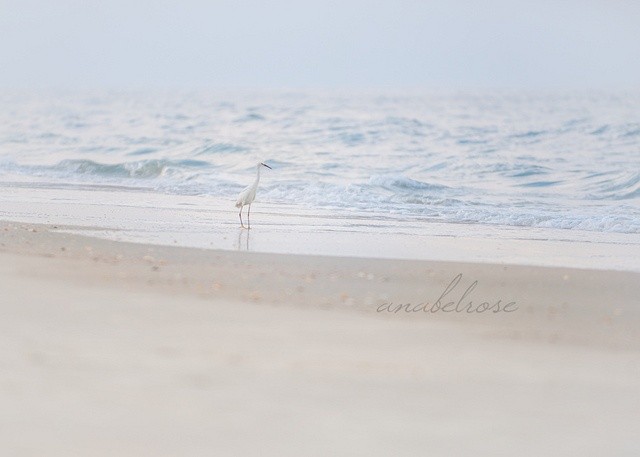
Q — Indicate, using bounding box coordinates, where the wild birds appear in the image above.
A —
[235,160,272,229]
